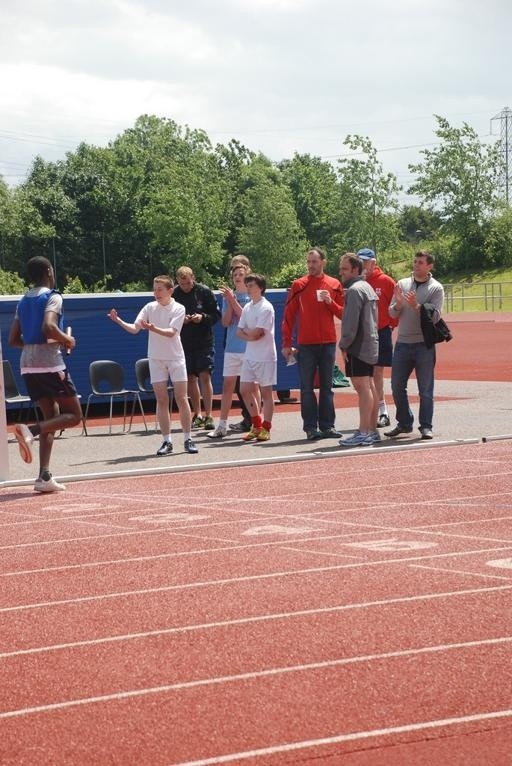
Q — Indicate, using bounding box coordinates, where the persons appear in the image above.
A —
[206,265,261,438]
[107,275,199,455]
[357,249,400,428]
[9,257,83,493]
[223,254,264,433]
[171,266,222,430]
[236,273,277,442]
[280,248,345,440]
[384,252,444,440]
[338,254,382,447]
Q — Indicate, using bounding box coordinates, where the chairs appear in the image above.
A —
[26,400,89,437]
[81,359,148,436]
[128,358,174,433]
[3,360,38,424]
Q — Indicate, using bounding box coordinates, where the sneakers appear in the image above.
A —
[422,428,433,439]
[157,441,173,455]
[244,424,263,441]
[205,415,215,430]
[14,424,34,463]
[185,440,198,453]
[230,421,249,430]
[308,429,324,440]
[384,424,412,436]
[258,428,271,440]
[192,416,204,429]
[323,426,342,437]
[206,426,227,438]
[340,432,374,446]
[377,414,391,427]
[34,475,66,493]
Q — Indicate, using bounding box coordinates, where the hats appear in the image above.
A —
[357,248,374,260]
